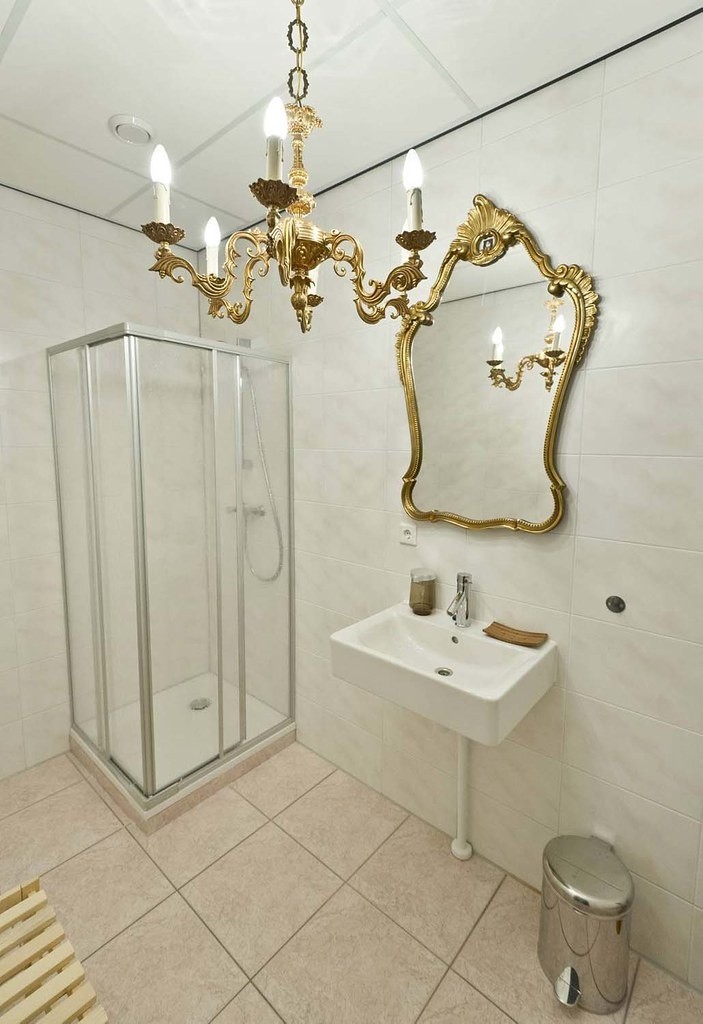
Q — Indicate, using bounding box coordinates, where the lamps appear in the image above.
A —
[142,1,434,334]
[486,294,569,392]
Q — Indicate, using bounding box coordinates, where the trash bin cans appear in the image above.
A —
[537,834,636,1016]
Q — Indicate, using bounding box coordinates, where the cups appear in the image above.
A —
[408,571,437,616]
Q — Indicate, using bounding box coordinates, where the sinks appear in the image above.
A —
[328,602,560,747]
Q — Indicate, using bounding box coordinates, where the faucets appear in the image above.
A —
[446,572,473,628]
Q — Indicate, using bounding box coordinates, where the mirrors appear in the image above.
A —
[397,193,601,534]
